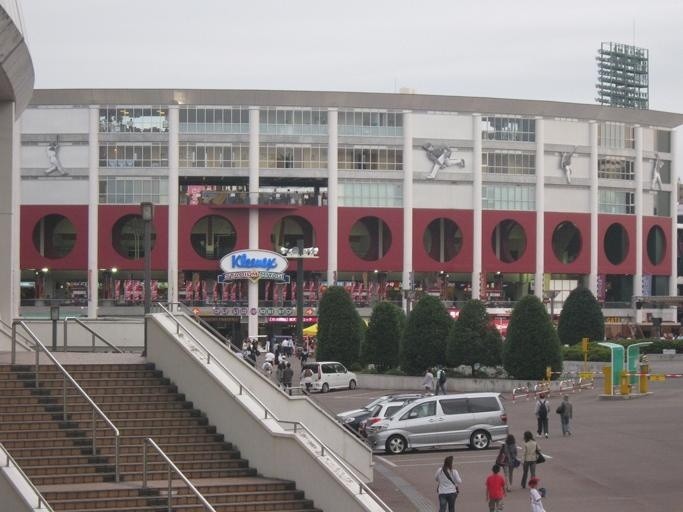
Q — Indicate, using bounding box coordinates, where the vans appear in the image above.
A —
[365,392,509,454]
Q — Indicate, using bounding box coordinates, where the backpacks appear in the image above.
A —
[440,370,446,384]
[539,400,547,418]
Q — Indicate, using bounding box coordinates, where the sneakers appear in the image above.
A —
[536,432,548,440]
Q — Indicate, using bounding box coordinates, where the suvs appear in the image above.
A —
[300,361,357,393]
[336,393,434,437]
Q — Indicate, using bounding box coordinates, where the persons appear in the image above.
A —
[435,365,448,394]
[534,392,550,439]
[527,480,545,512]
[501,433,518,491]
[434,455,463,511]
[235,337,313,395]
[561,394,573,436]
[420,368,433,392]
[520,429,540,488]
[484,464,508,511]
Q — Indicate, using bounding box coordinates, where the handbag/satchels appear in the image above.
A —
[495,444,508,466]
[536,450,546,464]
[556,406,565,414]
